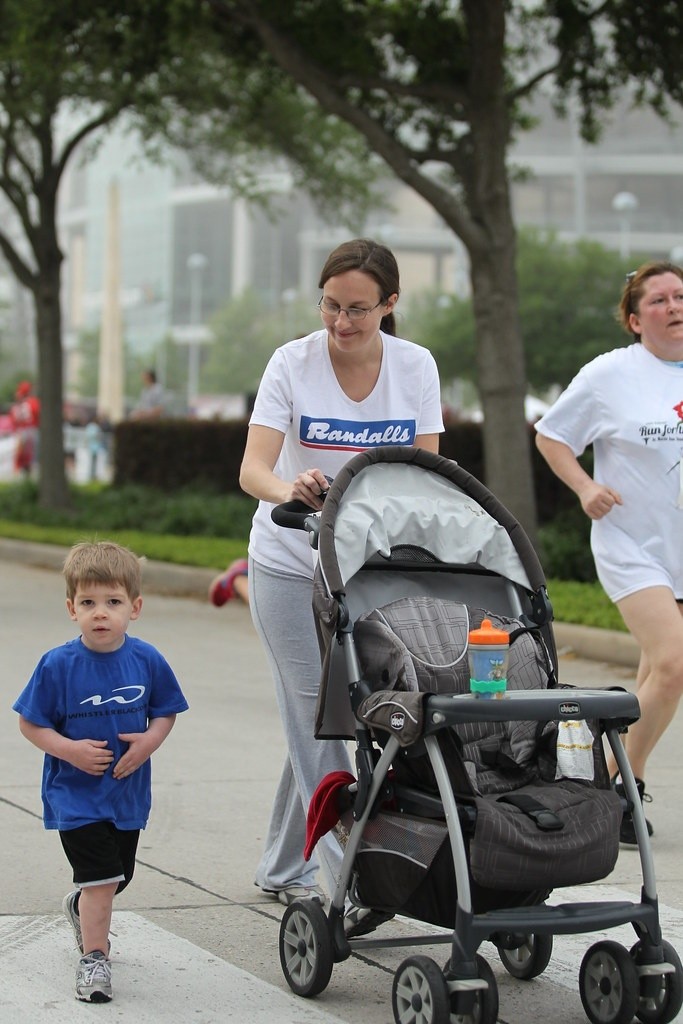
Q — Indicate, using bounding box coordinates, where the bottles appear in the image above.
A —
[467,619,509,701]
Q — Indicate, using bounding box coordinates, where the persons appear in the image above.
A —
[206,239,458,940]
[62,409,115,484]
[12,541,190,1003]
[533,264,683,849]
[10,379,39,481]
[129,366,169,418]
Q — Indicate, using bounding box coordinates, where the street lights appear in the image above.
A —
[189,251,205,416]
[613,188,638,258]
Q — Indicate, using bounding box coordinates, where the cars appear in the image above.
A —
[62,393,106,457]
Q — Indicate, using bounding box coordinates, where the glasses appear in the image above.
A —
[318,296,383,321]
[625,271,638,314]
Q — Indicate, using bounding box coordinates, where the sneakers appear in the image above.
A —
[610,770,653,849]
[342,903,397,939]
[75,949,113,1003]
[254,879,326,907]
[211,559,249,607]
[63,891,118,956]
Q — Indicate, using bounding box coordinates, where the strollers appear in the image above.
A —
[271,442,683,1024]
